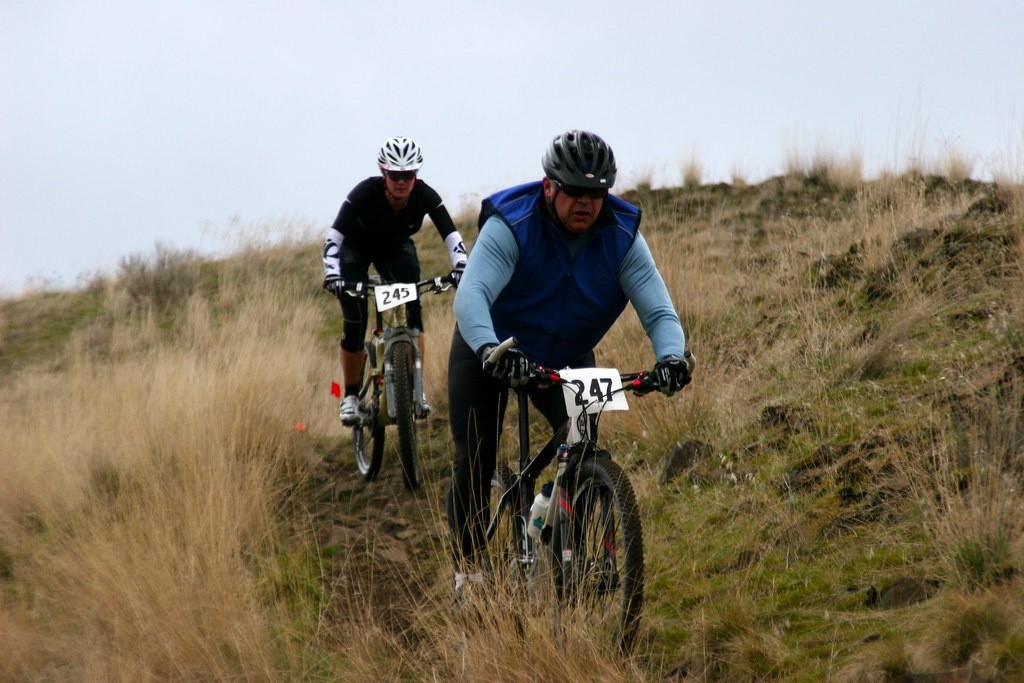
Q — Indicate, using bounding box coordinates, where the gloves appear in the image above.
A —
[449,266,465,284]
[323,275,345,297]
[633,357,689,399]
[480,346,530,389]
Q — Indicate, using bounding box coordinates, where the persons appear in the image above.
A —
[324,136,468,423]
[449,131,692,616]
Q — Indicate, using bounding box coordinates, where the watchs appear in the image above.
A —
[456,261,467,267]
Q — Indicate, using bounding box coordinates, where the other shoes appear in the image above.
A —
[565,561,619,590]
[451,577,488,624]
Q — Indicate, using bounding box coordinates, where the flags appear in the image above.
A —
[331,382,340,397]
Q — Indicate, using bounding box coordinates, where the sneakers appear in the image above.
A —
[339,394,359,421]
[419,392,431,417]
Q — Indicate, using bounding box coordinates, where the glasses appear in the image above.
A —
[551,177,608,199]
[385,171,417,182]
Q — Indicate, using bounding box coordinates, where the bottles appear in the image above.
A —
[528,481,554,539]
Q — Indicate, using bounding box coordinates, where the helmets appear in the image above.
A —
[377,136,422,173]
[541,128,616,189]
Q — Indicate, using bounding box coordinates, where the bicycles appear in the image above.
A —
[342,269,462,494]
[483,335,696,658]
